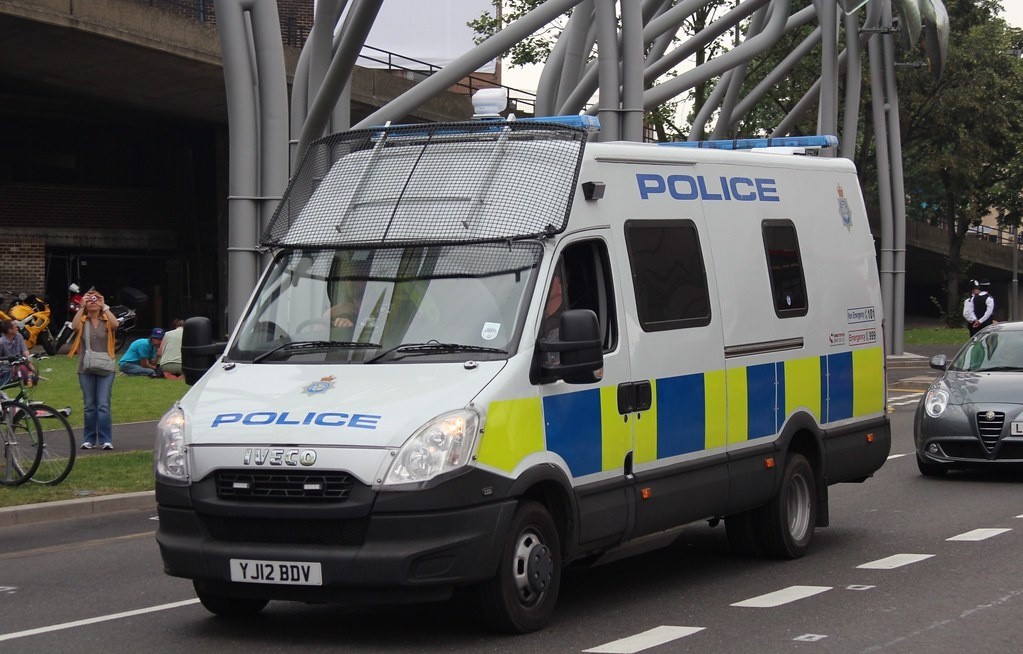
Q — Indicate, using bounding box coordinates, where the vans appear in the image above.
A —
[153,88,893,641]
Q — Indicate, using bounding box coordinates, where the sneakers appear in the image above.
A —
[80,442,96,449]
[102,442,114,449]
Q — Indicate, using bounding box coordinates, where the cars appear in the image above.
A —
[913,322,1022,478]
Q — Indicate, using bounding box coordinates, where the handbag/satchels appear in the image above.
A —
[82,350,114,376]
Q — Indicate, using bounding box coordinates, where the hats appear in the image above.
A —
[151,328,165,339]
[978,279,991,286]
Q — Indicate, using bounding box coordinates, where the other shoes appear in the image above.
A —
[178,376,184,380]
[164,372,177,380]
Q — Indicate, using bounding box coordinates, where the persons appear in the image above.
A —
[117,318,186,381]
[72,289,119,451]
[323,260,422,337]
[962,278,994,339]
[0,319,35,376]
[540,264,575,366]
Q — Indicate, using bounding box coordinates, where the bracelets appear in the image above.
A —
[80,305,85,308]
[102,305,110,312]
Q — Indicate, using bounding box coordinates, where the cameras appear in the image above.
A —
[89,296,97,302]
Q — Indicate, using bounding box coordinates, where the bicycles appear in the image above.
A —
[0,351,77,488]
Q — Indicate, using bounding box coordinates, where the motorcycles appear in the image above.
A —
[55,282,137,354]
[0,290,57,357]
